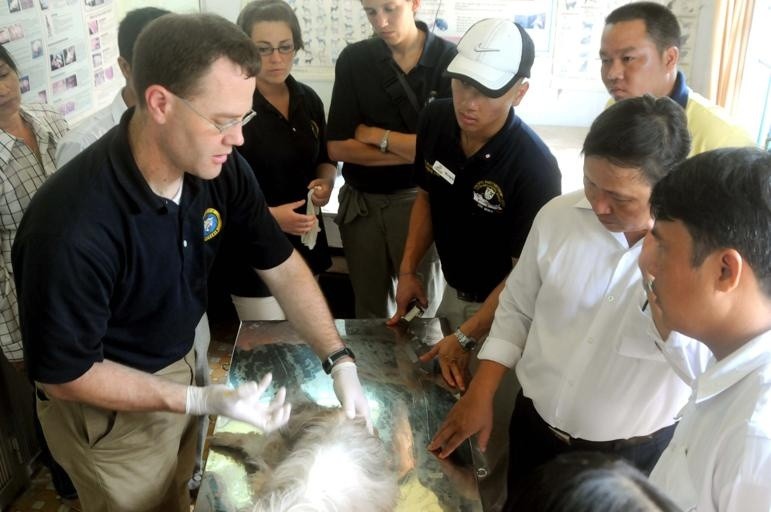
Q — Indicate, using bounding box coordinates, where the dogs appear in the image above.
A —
[206,402,401,512]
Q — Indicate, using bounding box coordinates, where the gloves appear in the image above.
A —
[330,362,373,434]
[185,372,291,433]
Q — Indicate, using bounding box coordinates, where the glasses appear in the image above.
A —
[180,98,257,133]
[256,44,294,56]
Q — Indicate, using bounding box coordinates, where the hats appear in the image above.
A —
[441,17,535,98]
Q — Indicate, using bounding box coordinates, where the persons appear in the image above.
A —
[1,0,771,512]
